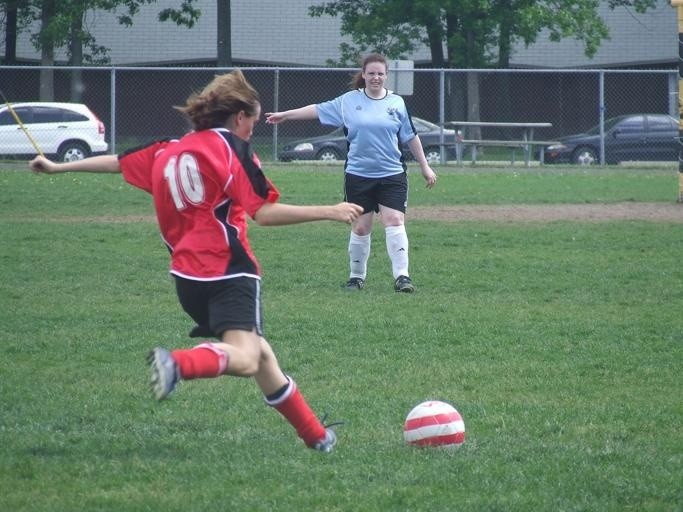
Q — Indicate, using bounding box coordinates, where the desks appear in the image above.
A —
[438,120,555,165]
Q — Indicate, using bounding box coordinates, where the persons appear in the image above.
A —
[28,66,364,456]
[264,54,437,293]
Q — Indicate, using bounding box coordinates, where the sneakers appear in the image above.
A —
[392,274,415,294]
[346,275,365,292]
[308,413,344,455]
[144,346,183,403]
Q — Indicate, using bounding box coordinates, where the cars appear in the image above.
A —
[0,102,108,163]
[535,113,680,166]
[279,113,463,162]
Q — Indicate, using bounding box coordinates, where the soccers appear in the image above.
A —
[403,401,466,450]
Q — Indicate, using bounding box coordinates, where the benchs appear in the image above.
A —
[428,137,559,167]
